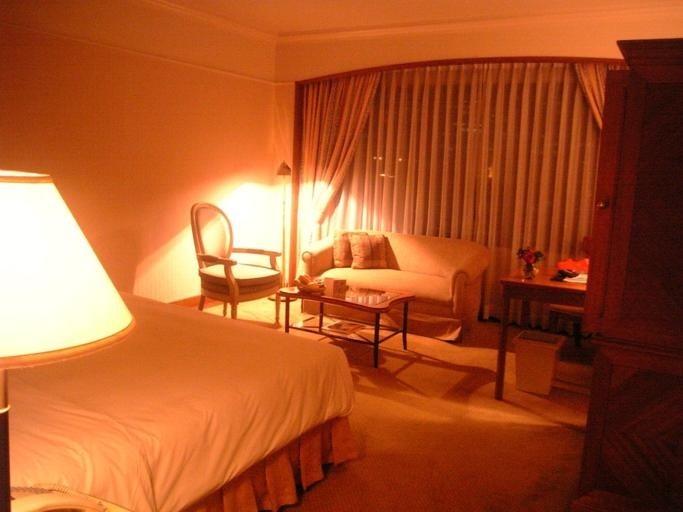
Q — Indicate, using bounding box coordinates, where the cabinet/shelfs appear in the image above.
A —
[577,34,682,505]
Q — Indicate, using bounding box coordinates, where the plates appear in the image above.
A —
[298,287,325,293]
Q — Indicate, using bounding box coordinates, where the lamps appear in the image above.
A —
[0,169,136,511]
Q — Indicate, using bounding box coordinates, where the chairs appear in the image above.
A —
[189,202,283,330]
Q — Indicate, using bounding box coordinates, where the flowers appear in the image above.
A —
[516,246,543,271]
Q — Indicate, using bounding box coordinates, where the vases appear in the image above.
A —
[520,264,539,280]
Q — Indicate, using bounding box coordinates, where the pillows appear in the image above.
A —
[330,230,387,269]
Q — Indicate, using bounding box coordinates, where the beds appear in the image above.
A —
[4,292,356,512]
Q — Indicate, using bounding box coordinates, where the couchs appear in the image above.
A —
[299,228,491,345]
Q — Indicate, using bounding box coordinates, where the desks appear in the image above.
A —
[494,267,586,401]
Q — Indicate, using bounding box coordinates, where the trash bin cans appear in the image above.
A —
[513,330,567,396]
